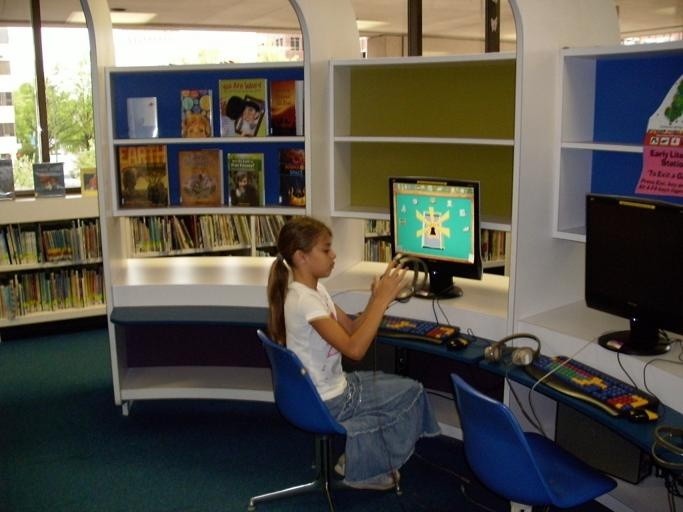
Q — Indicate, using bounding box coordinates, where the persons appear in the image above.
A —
[266,216,446,490]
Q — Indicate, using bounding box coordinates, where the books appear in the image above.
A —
[364,217,394,264]
[0,219,107,319]
[0,153,15,201]
[226,152,265,206]
[181,89,212,139]
[119,145,171,209]
[32,162,66,197]
[268,78,303,134]
[129,215,291,257]
[176,148,224,209]
[218,77,268,137]
[278,150,306,207]
[126,96,157,139]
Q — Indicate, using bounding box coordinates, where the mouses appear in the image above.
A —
[629,409,659,422]
[449,336,470,351]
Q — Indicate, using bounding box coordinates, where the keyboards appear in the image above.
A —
[350,311,460,346]
[533,356,659,417]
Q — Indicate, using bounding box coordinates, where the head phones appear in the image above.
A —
[379,253,427,303]
[484,333,541,366]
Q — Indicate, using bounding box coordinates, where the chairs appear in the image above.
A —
[246,329,404,512]
[449,371,618,512]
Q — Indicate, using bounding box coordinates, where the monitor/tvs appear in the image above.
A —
[585,193,683,356]
[389,176,482,299]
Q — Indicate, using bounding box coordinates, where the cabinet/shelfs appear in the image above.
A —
[104,61,306,217]
[328,51,523,234]
[0,196,510,344]
[549,41,683,244]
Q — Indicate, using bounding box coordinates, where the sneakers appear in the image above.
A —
[334,453,345,476]
[342,469,400,490]
